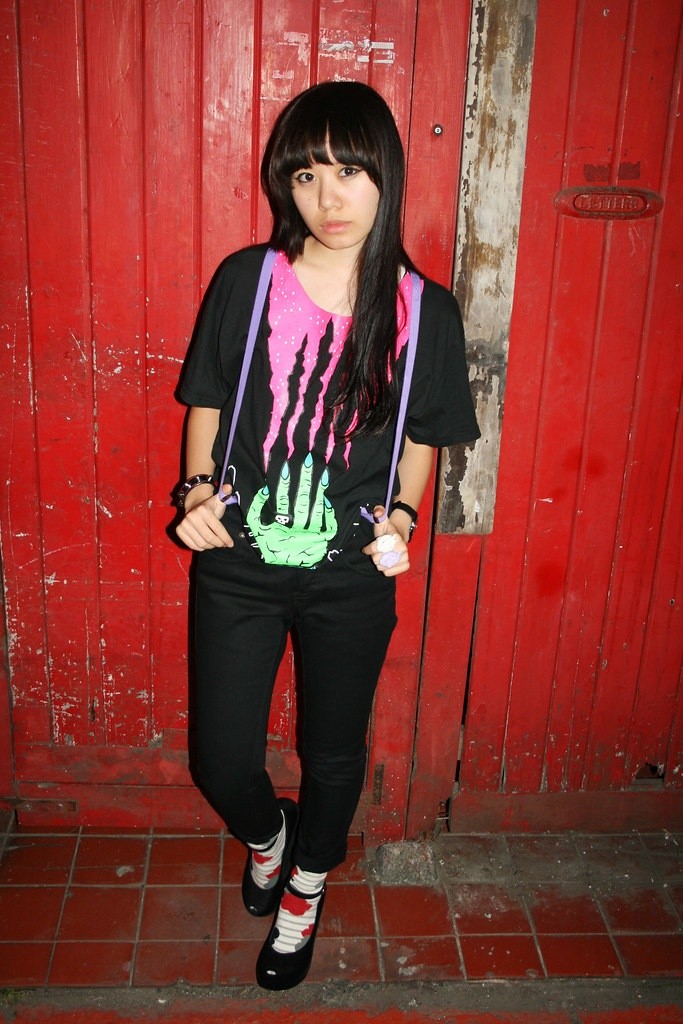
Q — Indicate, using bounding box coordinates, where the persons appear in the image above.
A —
[168,79,482,988]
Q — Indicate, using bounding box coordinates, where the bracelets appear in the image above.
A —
[174,473,219,509]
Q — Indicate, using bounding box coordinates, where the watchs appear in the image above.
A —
[383,499,420,541]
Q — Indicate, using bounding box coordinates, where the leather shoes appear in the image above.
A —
[241,797,326,990]
